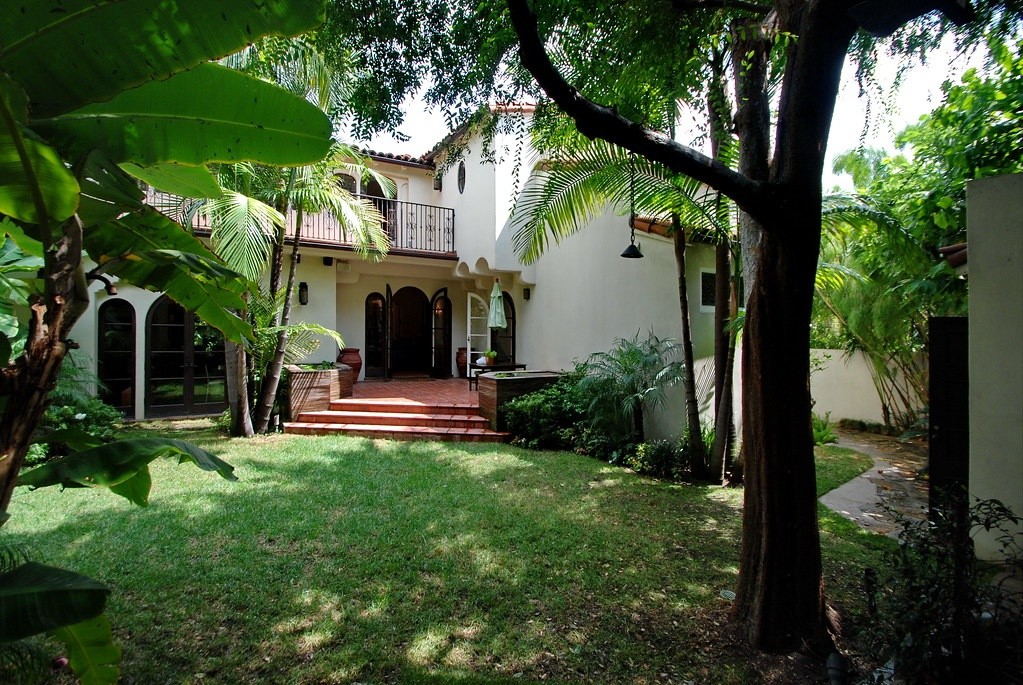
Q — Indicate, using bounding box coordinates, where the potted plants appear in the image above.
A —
[486,351,497,365]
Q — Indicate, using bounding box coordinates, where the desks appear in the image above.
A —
[469,361,526,391]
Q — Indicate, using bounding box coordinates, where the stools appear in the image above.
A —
[474,371,489,390]
